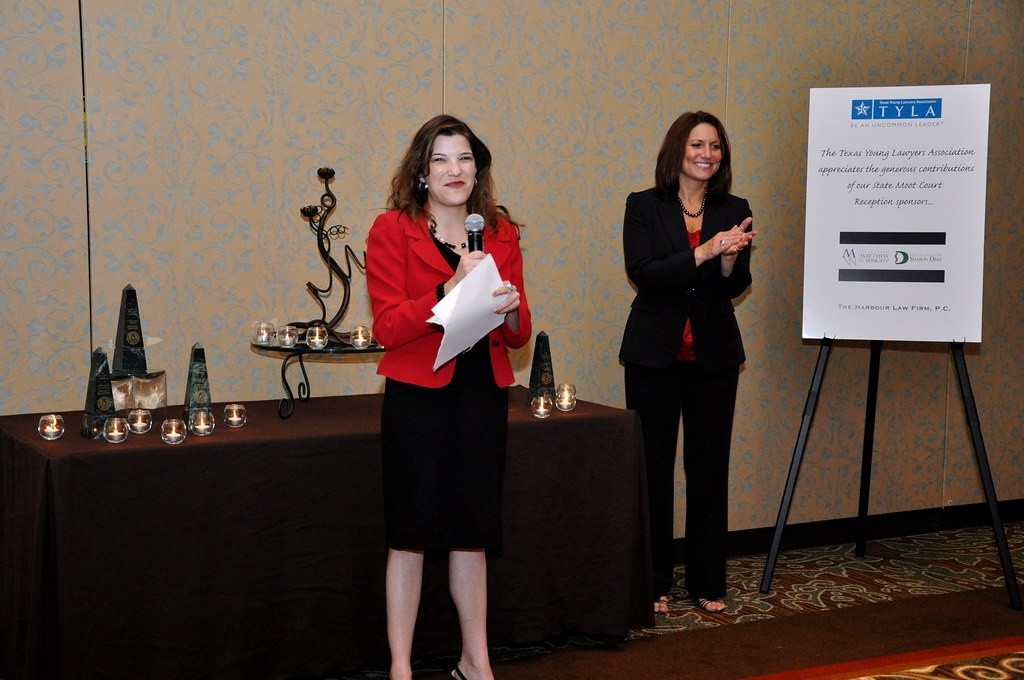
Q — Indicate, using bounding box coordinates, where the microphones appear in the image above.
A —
[464,214,485,253]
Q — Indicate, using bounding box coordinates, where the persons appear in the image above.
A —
[619,111,757,616]
[366,115,532,680]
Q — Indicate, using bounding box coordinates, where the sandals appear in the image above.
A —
[699,598,726,613]
[655,595,669,613]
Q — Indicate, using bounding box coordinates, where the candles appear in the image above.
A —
[127,410,152,433]
[103,418,128,443]
[277,326,298,347]
[161,419,188,445]
[189,412,215,436]
[253,322,275,344]
[350,326,371,349]
[532,396,552,418]
[556,391,577,412]
[223,404,246,427]
[37,414,65,439]
[556,384,576,399]
[307,326,329,349]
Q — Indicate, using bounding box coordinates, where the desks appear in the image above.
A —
[0,386,655,680]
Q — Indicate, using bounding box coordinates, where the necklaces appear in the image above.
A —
[678,192,707,217]
[427,222,469,248]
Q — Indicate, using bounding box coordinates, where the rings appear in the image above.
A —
[720,240,724,246]
[736,245,743,251]
[740,225,745,230]
[506,283,513,293]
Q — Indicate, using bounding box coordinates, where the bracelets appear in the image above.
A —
[437,282,445,302]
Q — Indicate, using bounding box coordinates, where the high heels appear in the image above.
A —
[450,660,467,680]
